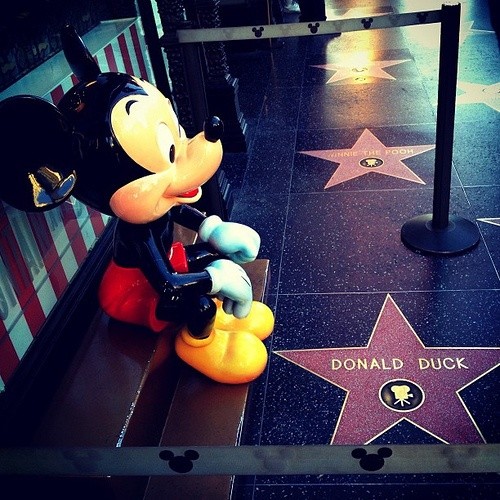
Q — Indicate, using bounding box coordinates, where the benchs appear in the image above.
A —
[43,257,272,500]
